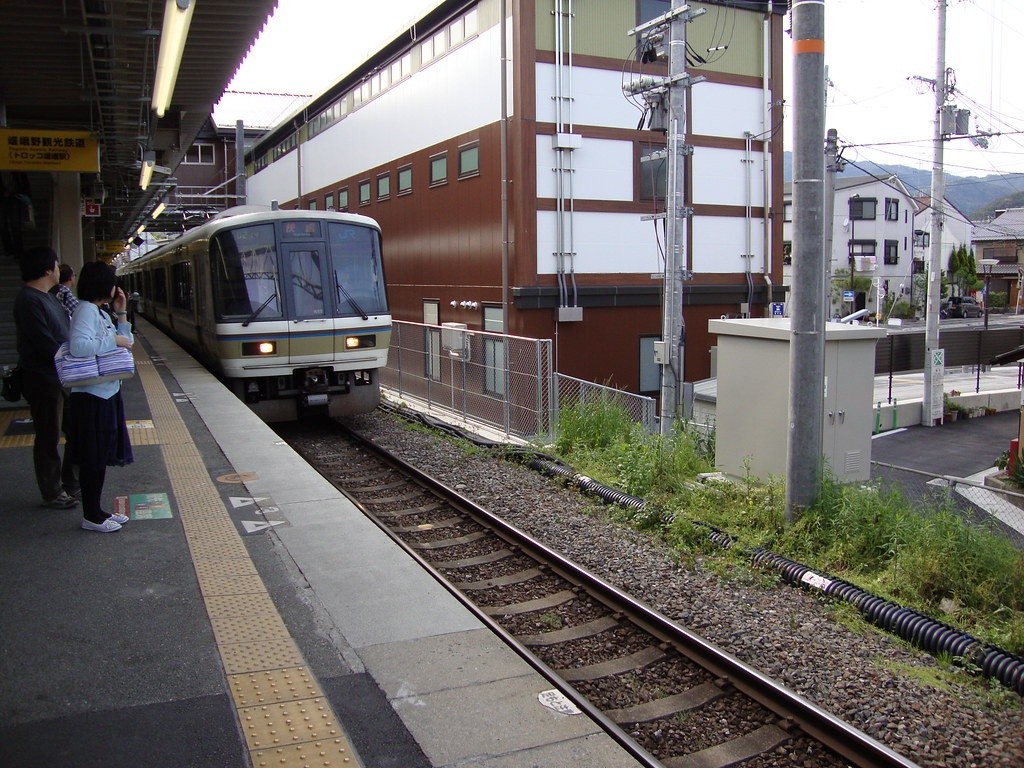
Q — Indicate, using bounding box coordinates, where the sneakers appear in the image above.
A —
[43,491,80,509]
[72,489,82,500]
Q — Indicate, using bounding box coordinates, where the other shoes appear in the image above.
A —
[82,518,122,532]
[109,513,129,523]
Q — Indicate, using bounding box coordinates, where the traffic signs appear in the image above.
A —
[843,291,854,301]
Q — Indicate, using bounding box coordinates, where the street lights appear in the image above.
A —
[978,259,1001,331]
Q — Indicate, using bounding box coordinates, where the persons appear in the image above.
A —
[14,247,140,531]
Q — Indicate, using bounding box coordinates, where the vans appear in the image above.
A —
[948,297,983,318]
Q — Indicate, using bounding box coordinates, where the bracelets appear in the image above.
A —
[116,311,127,315]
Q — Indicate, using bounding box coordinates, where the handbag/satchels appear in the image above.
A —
[1,368,19,402]
[53,305,135,387]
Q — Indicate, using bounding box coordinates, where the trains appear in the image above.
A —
[116,200,393,423]
